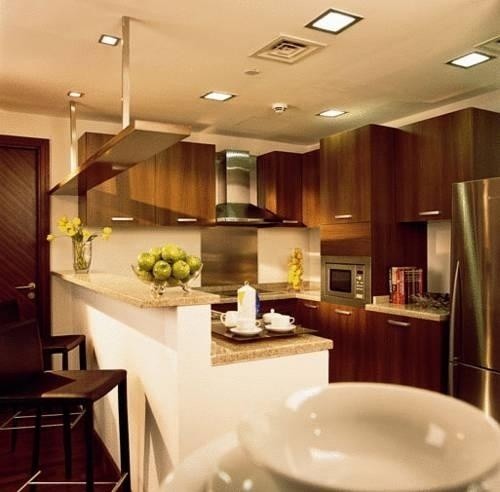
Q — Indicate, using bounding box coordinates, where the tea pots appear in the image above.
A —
[238,280,262,328]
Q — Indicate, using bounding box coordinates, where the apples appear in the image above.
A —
[186,255,200,273]
[178,249,185,260]
[138,252,156,271]
[153,261,171,279]
[173,260,188,279]
[162,244,178,262]
[150,248,163,261]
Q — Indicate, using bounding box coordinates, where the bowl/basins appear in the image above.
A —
[238,382,500,488]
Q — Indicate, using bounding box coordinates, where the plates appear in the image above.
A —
[133,264,206,295]
[266,323,298,332]
[230,326,264,334]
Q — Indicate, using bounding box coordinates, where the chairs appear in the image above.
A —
[3,298,87,451]
[0,318,129,492]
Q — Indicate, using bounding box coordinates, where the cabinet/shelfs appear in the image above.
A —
[296,297,361,384]
[75,130,217,231]
[362,304,449,395]
[390,107,500,222]
[320,125,429,295]
[254,150,301,222]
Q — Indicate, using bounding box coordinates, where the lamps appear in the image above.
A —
[272,102,289,114]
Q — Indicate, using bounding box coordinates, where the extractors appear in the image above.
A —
[214,149,280,228]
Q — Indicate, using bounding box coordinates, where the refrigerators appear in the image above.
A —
[446,177,500,426]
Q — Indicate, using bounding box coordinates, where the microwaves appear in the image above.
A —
[318,255,372,309]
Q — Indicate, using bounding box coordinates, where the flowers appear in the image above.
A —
[47,215,113,272]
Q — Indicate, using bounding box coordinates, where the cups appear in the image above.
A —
[219,312,238,328]
[266,314,294,327]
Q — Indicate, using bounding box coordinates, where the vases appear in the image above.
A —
[72,240,93,274]
